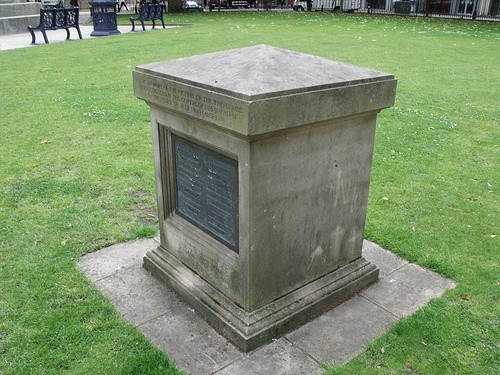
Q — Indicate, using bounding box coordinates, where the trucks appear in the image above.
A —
[292,0,362,13]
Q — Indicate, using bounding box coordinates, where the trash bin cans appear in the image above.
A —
[88,0,122,36]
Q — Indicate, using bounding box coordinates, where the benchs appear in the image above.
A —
[129,5,166,32]
[28,8,83,44]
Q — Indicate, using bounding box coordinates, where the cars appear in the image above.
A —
[185,1,198,8]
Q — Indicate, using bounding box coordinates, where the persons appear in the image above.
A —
[70,0,79,10]
